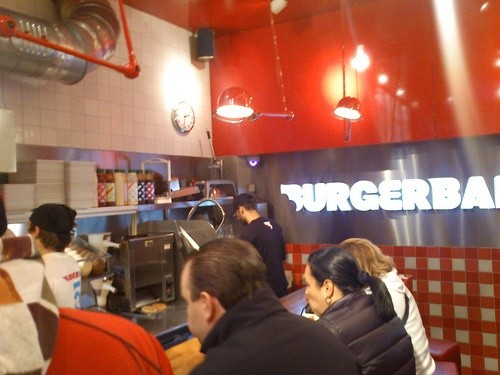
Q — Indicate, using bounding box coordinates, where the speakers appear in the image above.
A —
[194,28,216,60]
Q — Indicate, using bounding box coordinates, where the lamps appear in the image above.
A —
[174,197,226,250]
[333,0,363,144]
[212,0,295,124]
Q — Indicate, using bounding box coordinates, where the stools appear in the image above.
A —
[428,337,461,367]
[432,361,459,375]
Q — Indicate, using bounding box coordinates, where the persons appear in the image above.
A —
[28,202,99,310]
[229,192,289,298]
[0,236,175,375]
[178,238,360,375]
[301,246,416,375]
[337,237,437,375]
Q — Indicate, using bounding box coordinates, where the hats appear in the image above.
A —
[230,194,256,218]
[30,203,77,233]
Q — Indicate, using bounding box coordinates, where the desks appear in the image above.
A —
[165,287,320,375]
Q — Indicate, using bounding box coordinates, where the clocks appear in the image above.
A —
[171,102,195,133]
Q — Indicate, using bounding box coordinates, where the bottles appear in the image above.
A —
[146,170,155,204]
[136,169,146,204]
[105,169,115,206]
[114,169,138,206]
[97,168,107,207]
[172,175,195,202]
